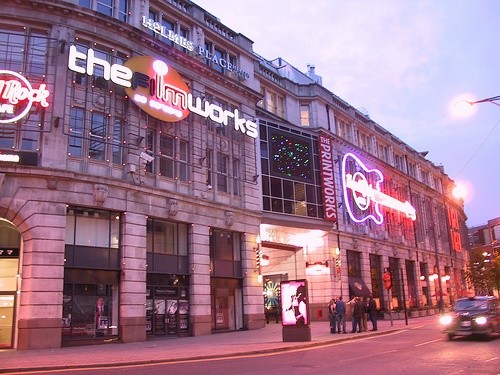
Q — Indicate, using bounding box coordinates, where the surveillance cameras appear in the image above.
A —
[139,152,156,162]
[207,185,212,191]
[129,165,136,173]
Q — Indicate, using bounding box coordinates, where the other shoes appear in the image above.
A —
[338,331,340,333]
[358,331,362,333]
[350,330,355,333]
[343,332,347,334]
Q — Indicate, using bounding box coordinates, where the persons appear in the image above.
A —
[328,293,379,334]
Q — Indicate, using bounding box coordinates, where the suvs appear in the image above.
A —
[440,295,500,342]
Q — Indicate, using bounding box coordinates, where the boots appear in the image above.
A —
[331,328,335,333]
